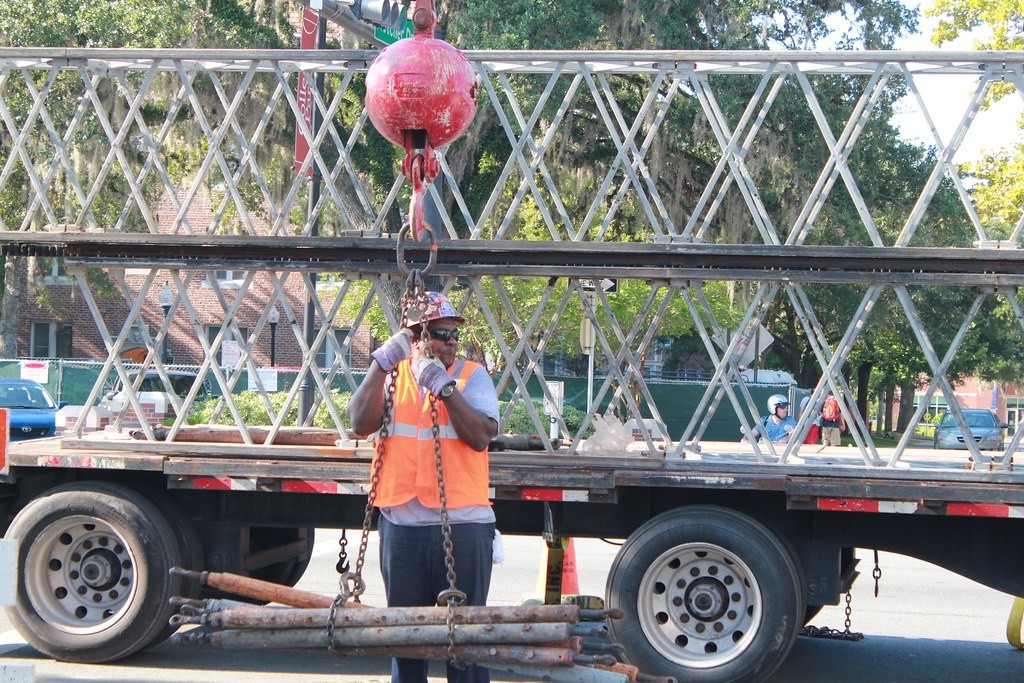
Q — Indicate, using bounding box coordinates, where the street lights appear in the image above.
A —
[159,281,174,363]
[268,306,280,367]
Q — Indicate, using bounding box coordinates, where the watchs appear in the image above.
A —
[435,381,457,399]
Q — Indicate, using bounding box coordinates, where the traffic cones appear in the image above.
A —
[559,535,581,605]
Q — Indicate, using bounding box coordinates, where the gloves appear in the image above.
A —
[372,329,416,372]
[409,356,457,398]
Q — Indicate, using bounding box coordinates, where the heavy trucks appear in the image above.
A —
[0,44,1024,682]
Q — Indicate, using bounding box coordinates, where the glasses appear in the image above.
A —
[417,329,462,341]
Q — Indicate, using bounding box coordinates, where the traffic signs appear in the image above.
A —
[373,14,414,45]
[568,276,620,294]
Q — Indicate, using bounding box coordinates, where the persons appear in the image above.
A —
[819,390,845,446]
[799,387,821,444]
[740,394,800,445]
[347,291,500,683]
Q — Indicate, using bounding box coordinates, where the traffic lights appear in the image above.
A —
[360,0,409,31]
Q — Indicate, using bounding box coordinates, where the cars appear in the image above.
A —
[933,409,1009,451]
[1,378,70,442]
[109,368,212,418]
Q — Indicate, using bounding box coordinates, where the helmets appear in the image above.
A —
[767,394,789,415]
[403,291,465,326]
